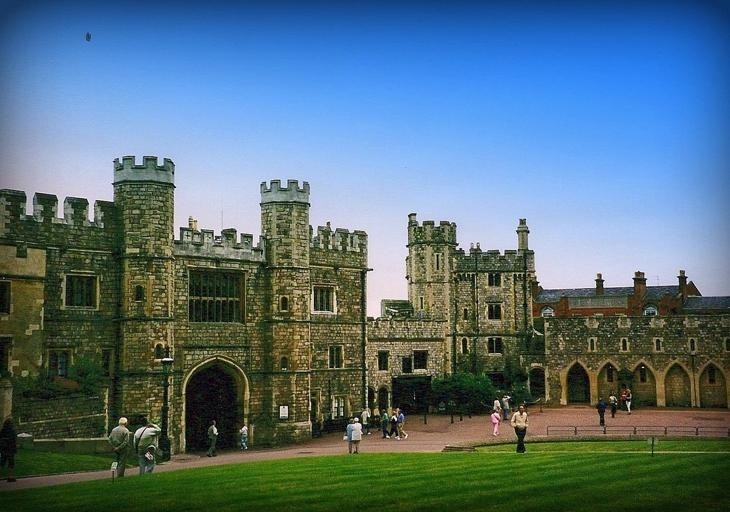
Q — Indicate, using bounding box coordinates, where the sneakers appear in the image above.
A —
[208,453,217,457]
[387,434,409,441]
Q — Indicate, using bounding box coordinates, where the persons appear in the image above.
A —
[510,404,529,454]
[0,417,18,483]
[625,389,633,414]
[608,391,618,418]
[493,396,501,413]
[344,405,411,454]
[489,409,500,436]
[620,387,626,408]
[502,394,511,420]
[206,420,219,457]
[107,416,130,478]
[596,395,608,427]
[132,417,162,475]
[237,423,249,453]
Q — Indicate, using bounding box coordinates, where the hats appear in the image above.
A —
[354,418,359,422]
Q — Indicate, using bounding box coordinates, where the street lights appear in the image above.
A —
[158,346,176,459]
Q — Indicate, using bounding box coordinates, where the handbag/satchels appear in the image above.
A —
[342,435,348,441]
[145,451,154,462]
